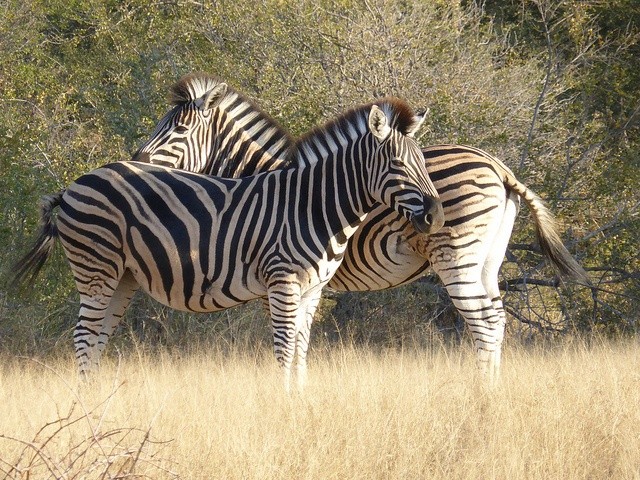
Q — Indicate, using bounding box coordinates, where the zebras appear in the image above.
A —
[11,98,447,413]
[130,73,591,396]
